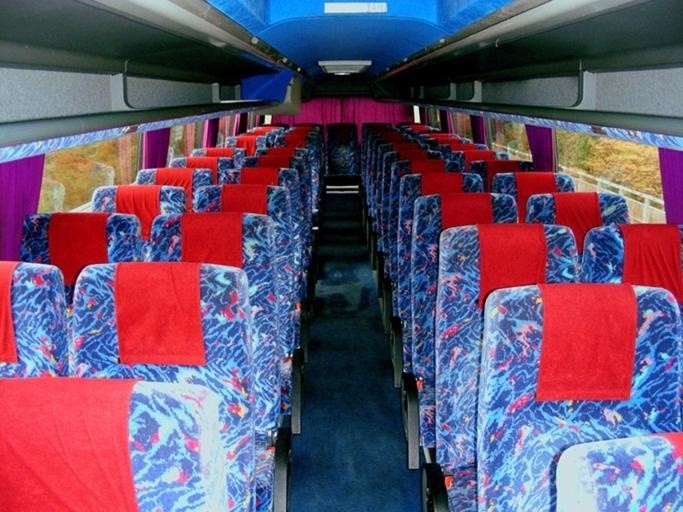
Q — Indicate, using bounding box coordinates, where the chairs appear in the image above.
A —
[65,258,262,512]
[145,210,307,472]
[18,204,145,295]
[87,182,189,243]
[552,427,681,512]
[398,189,524,471]
[191,181,299,418]
[520,190,632,258]
[326,125,360,180]
[220,167,305,300]
[134,166,212,191]
[568,221,682,305]
[410,221,584,509]
[1,256,71,378]
[359,120,577,388]
[167,121,328,254]
[3,372,233,512]
[469,280,682,510]
